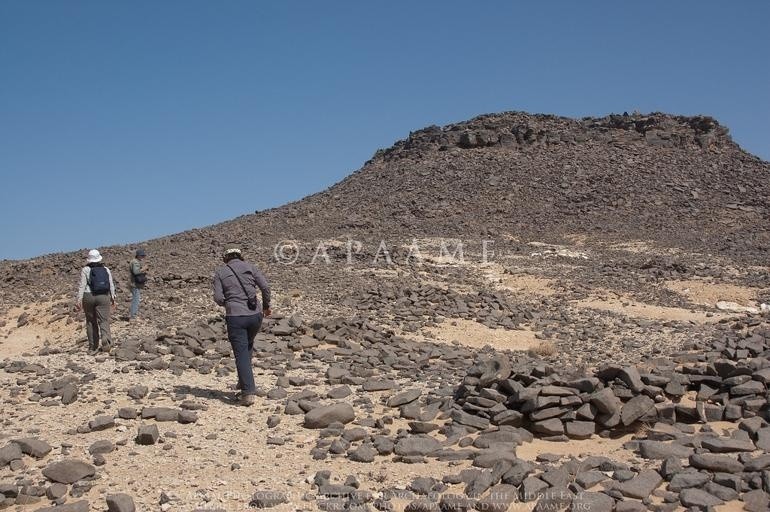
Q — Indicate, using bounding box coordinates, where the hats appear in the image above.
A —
[222,243,241,256]
[135,249,146,258]
[86,248,103,263]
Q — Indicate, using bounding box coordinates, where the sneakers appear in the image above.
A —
[89,344,111,353]
[236,379,255,406]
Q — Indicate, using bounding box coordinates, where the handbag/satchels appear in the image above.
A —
[134,273,147,283]
[86,264,110,294]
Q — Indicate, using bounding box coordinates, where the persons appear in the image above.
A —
[76,249,117,353]
[212,242,273,406]
[127,249,150,319]
